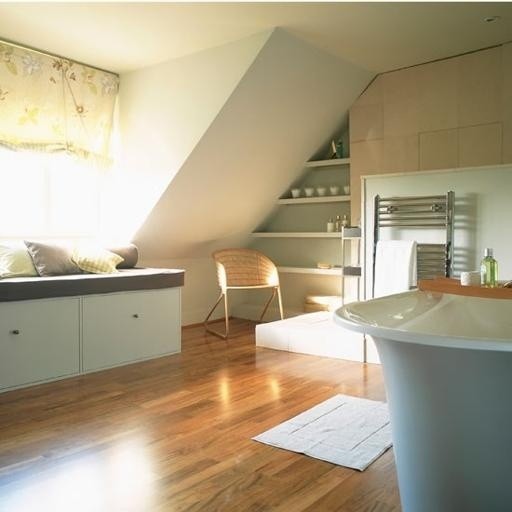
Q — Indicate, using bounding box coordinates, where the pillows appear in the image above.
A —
[104,241,137,271]
[74,244,125,274]
[1,241,37,280]
[23,239,82,277]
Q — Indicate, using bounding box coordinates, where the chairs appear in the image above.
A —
[201,249,285,339]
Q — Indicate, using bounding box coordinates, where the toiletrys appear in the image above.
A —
[333,215,341,232]
[341,214,349,227]
[326,217,335,232]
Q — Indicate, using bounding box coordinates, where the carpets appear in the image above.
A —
[251,392,393,474]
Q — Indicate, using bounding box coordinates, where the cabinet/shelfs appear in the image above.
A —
[2,294,81,393]
[230,154,352,325]
[81,285,182,376]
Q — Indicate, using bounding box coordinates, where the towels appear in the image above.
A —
[372,239,417,299]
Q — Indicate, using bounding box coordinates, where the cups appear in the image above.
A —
[316,188,328,196]
[290,187,314,197]
[460,270,479,285]
[330,187,340,195]
[344,186,349,193]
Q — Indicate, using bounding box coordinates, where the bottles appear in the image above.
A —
[326,214,348,233]
[335,139,343,159]
